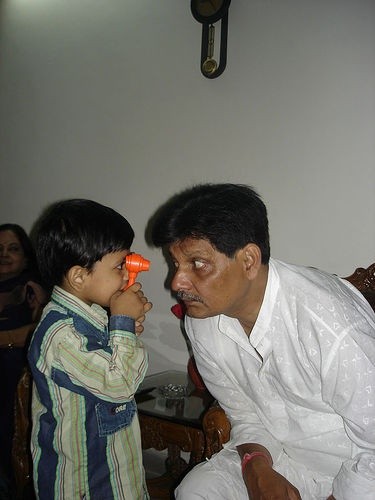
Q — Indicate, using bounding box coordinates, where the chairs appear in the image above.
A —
[11,370,34,500]
[201,261,375,460]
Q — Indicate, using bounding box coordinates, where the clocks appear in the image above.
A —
[191,0,231,79]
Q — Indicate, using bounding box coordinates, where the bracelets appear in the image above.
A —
[242,451,271,478]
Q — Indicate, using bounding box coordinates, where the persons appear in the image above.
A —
[27,198,153,500]
[150,183,375,500]
[0,223,48,500]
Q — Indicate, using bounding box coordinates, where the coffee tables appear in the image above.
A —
[134,368,210,477]
